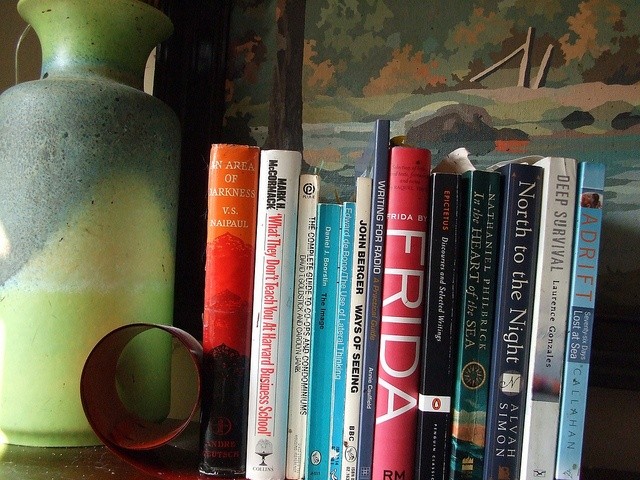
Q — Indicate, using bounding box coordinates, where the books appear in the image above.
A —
[484,163,545,479]
[342,177,372,480]
[372,145,433,480]
[199,141,260,480]
[449,169,503,480]
[305,203,341,480]
[246,148,302,480]
[354,119,390,480]
[521,155,581,479]
[553,161,608,479]
[284,173,334,480]
[329,200,355,480]
[413,171,461,480]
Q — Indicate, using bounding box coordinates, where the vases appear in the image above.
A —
[3,1,186,446]
